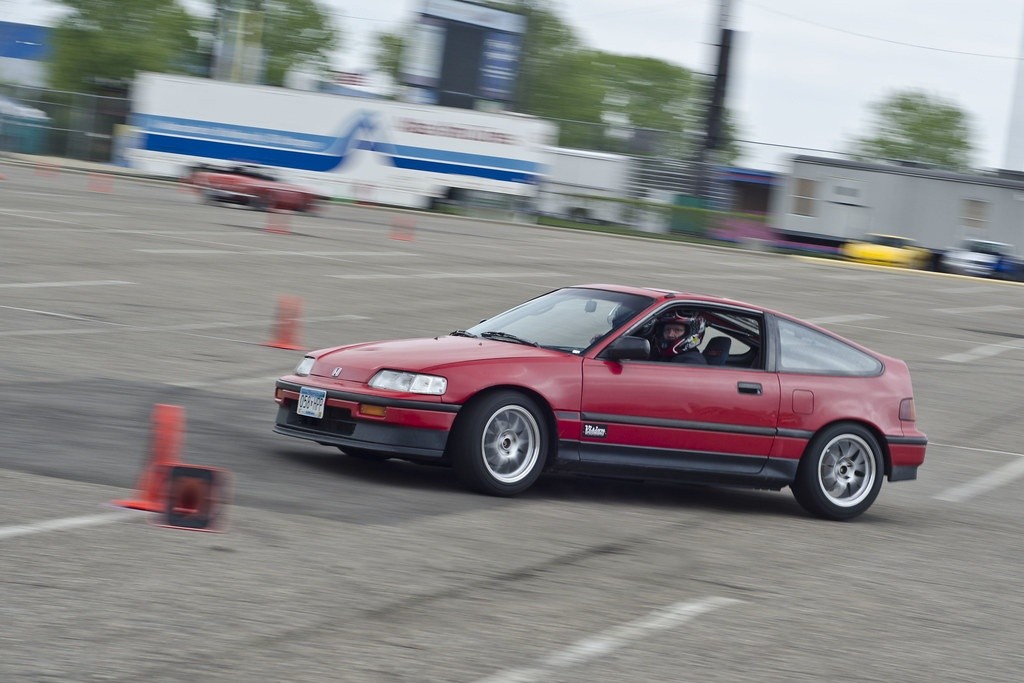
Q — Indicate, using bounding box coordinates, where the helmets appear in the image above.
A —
[659,306,707,359]
[612,301,657,339]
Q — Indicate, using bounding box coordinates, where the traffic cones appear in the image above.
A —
[262,301,306,351]
[153,462,225,530]
[113,404,184,513]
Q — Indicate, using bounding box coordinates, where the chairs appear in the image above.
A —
[701,336,731,367]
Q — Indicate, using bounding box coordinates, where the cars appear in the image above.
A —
[844,233,930,273]
[943,238,1018,280]
[267,281,926,521]
[193,164,317,217]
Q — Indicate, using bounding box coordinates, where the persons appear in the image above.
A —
[591,300,657,345]
[653,308,706,365]
